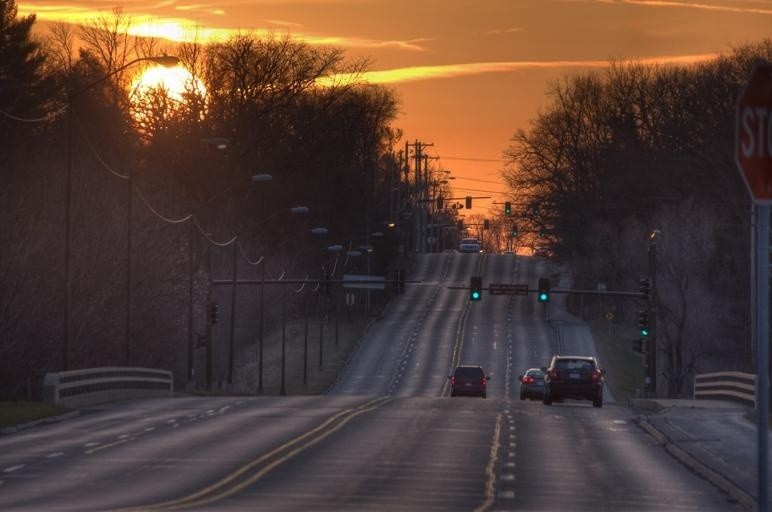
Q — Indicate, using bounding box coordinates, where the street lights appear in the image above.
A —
[59,55,384,398]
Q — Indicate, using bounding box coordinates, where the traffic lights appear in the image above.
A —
[537,278,550,304]
[638,309,649,337]
[470,276,482,302]
[504,202,512,216]
[512,225,518,237]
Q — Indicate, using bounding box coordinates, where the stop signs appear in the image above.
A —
[733,60,772,206]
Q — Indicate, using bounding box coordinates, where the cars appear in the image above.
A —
[447,364,490,400]
[459,237,481,253]
[541,354,606,409]
[518,367,545,401]
